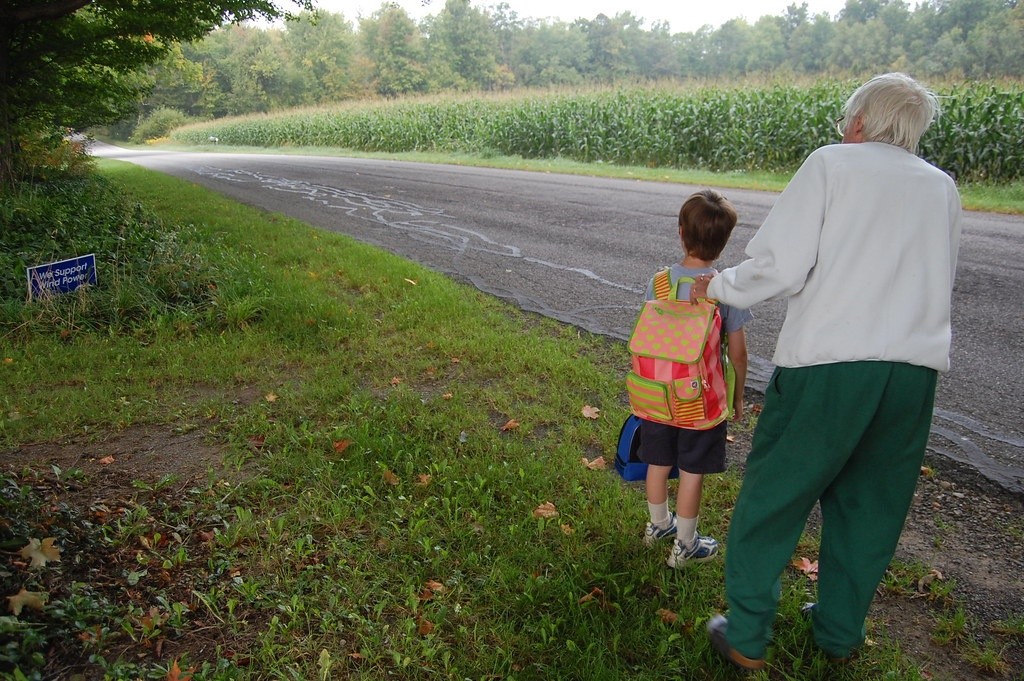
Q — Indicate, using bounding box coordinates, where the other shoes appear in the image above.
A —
[804,602,848,664]
[708,616,766,671]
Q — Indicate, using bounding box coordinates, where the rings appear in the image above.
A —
[694,288,696,292]
[701,273,705,277]
[700,276,703,280]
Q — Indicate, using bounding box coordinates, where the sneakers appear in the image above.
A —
[667,532,718,568]
[643,511,677,546]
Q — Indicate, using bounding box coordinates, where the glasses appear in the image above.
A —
[836,116,846,137]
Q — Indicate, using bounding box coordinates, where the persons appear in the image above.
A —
[688,74,962,671]
[626,189,755,569]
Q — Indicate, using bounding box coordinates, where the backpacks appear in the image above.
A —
[626,268,736,430]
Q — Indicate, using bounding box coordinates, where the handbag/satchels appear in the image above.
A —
[615,414,679,481]
